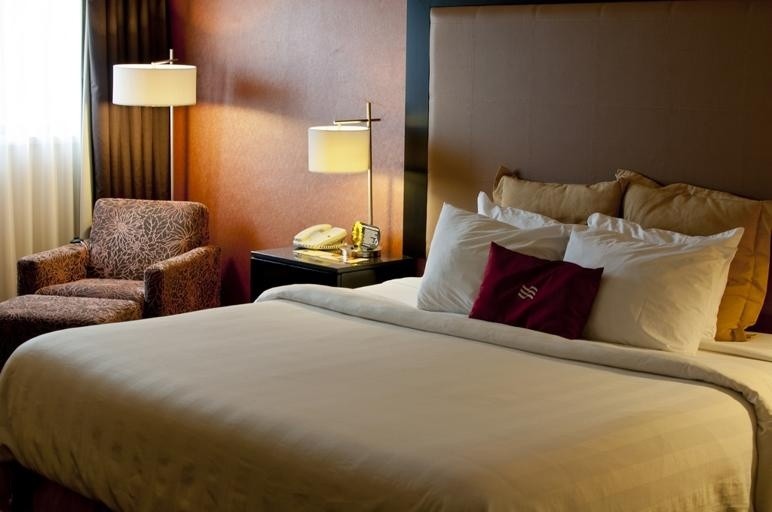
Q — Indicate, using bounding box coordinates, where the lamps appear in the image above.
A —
[112,48,197,201]
[308,101,382,257]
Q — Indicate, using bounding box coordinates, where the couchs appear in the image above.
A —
[15,197,222,318]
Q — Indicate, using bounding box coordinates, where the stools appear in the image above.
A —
[0,295,142,342]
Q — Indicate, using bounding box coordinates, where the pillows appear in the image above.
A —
[467,241,605,340]
[588,212,746,340]
[475,190,561,229]
[417,202,568,313]
[492,165,629,226]
[615,167,771,343]
[565,223,737,360]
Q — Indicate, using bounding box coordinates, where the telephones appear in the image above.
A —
[293,224,348,250]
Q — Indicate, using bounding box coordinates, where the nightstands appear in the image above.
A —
[251,248,412,302]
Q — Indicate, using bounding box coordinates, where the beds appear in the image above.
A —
[1,275,770,511]
